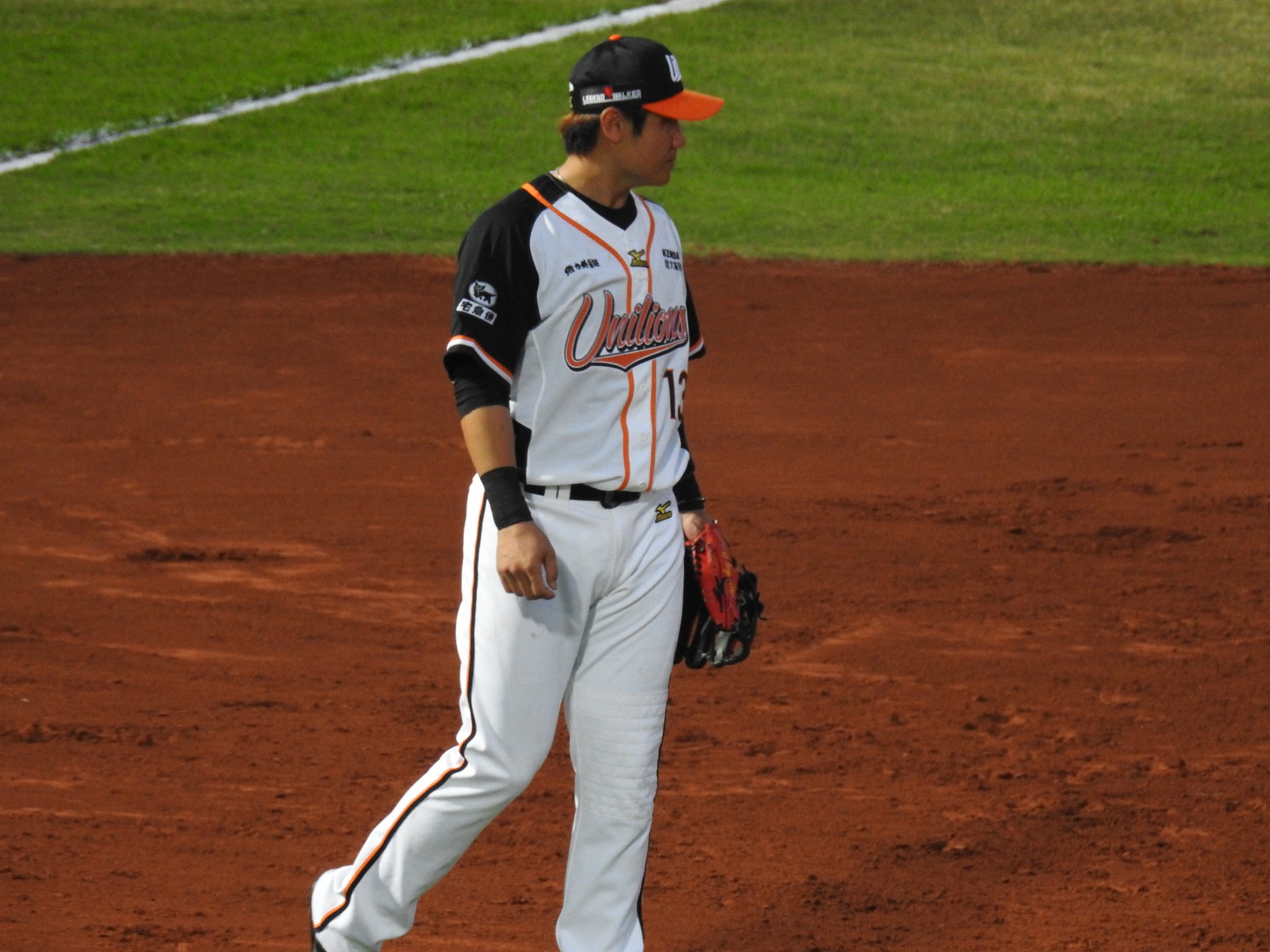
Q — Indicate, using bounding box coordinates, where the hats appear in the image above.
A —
[569,35,724,120]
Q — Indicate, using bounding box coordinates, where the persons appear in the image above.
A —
[309,32,740,952]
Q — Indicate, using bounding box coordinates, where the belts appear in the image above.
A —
[524,477,642,510]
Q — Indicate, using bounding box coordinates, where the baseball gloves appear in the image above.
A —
[671,522,762,669]
